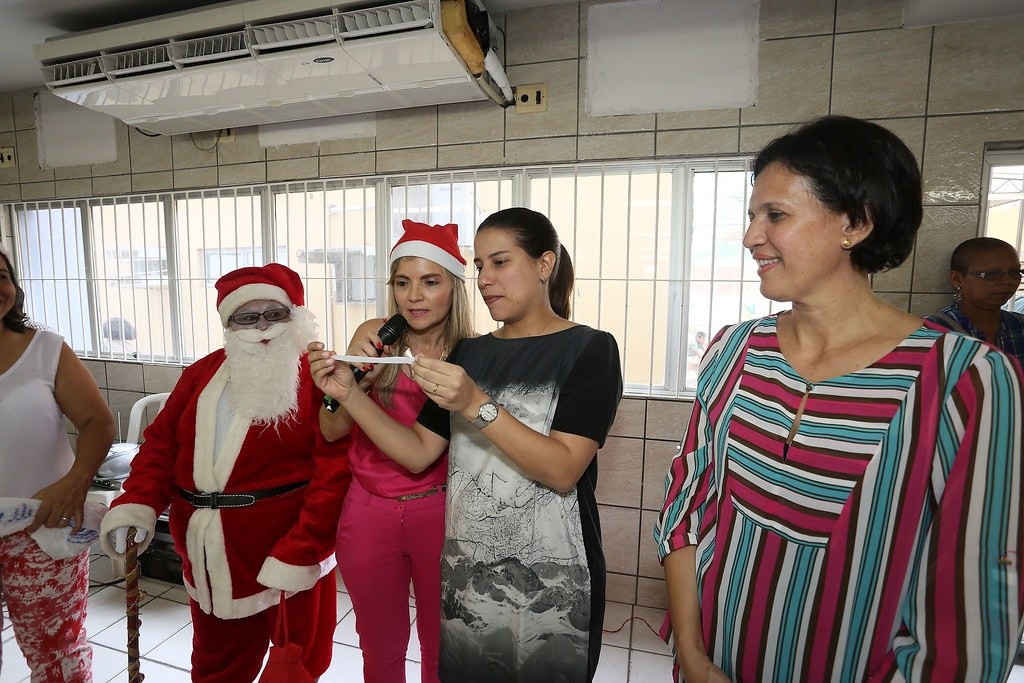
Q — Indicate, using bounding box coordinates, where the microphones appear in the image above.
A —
[322,313,407,412]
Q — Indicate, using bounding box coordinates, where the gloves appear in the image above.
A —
[109,525,148,554]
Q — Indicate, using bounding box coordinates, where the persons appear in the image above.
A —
[305,206,623,683]
[925,233,1023,373]
[0,244,120,683]
[316,217,481,683]
[650,115,1024,683]
[99,261,353,683]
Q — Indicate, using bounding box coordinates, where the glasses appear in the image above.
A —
[962,269,1024,281]
[228,308,289,325]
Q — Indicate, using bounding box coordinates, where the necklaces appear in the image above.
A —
[406,328,449,381]
[503,313,559,340]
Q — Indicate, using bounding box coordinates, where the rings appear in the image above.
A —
[61,517,71,521]
[432,384,440,395]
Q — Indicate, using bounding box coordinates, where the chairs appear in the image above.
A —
[85,392,171,579]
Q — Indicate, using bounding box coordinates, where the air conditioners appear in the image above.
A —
[33,0,516,135]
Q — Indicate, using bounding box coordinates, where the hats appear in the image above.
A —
[216,263,304,329]
[390,219,467,283]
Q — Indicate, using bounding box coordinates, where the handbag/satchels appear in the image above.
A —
[0,495,108,560]
[257,590,315,682]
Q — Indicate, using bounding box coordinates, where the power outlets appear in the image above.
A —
[212,128,236,143]
[516,83,548,114]
[0,147,16,168]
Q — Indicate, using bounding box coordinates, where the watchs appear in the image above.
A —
[467,396,500,430]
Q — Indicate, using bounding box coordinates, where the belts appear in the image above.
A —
[175,479,311,507]
[397,483,447,501]
